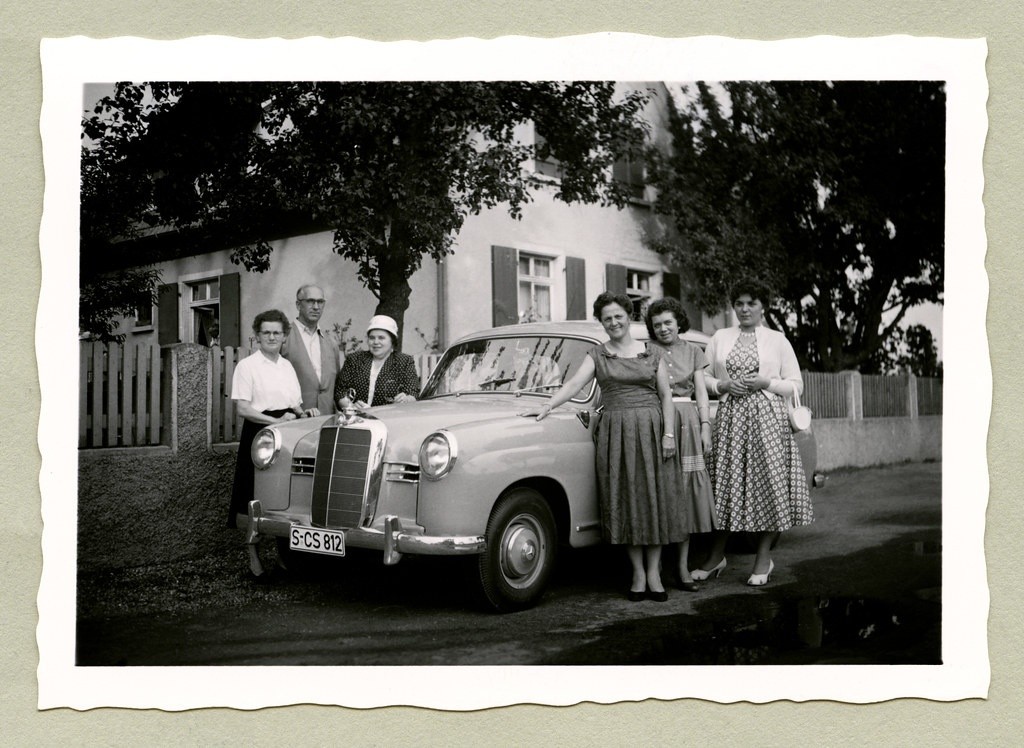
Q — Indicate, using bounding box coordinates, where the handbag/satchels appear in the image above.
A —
[787,383,812,433]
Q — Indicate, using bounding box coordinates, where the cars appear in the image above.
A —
[235,317,825,614]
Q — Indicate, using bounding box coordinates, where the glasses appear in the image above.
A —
[298,298,326,304]
[258,330,285,338]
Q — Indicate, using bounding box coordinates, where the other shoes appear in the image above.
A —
[249,567,266,584]
[678,570,700,591]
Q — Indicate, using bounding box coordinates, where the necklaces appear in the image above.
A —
[740,331,756,338]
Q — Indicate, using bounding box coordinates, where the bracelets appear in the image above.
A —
[700,419,712,426]
[541,401,553,413]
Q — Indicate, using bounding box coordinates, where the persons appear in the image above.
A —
[642,295,715,593]
[206,322,219,347]
[690,275,817,588]
[279,284,342,419]
[332,314,421,412]
[222,309,321,588]
[518,288,690,603]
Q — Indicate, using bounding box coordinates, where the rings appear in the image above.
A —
[709,447,713,451]
[745,385,749,389]
[670,453,673,456]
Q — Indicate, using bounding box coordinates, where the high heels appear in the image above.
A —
[747,557,774,585]
[628,574,647,601]
[647,573,668,602]
[689,556,727,580]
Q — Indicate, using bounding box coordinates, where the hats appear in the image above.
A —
[366,315,399,337]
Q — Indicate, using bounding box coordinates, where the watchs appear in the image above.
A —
[663,433,675,439]
